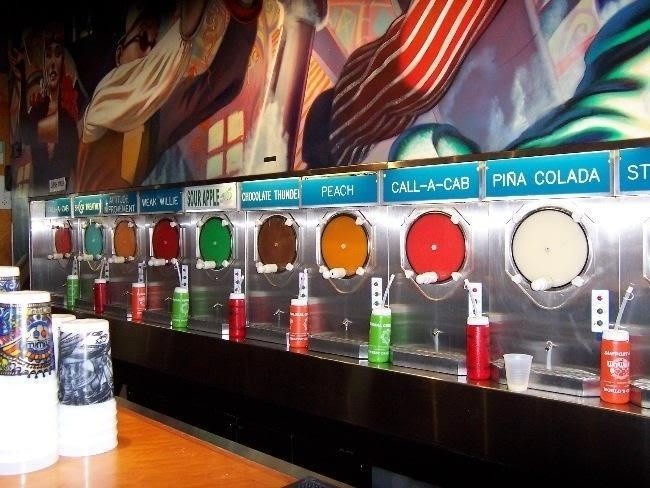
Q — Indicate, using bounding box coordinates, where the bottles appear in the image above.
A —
[229,293,244,338]
[600,329,630,402]
[67,275,80,308]
[95,279,106,314]
[172,287,189,327]
[368,308,391,363]
[466,316,490,380]
[132,283,145,320]
[289,299,308,347]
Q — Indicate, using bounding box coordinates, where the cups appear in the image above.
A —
[0,265,120,476]
[503,354,533,391]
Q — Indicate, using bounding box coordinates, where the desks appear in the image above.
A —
[0,391,347,487]
